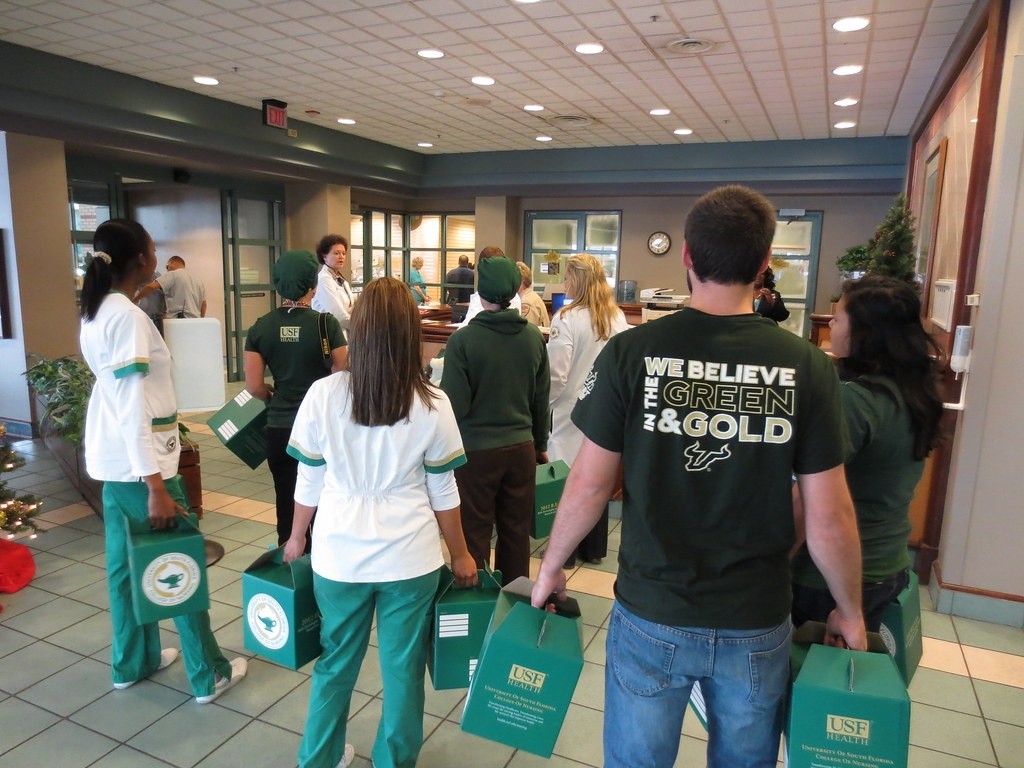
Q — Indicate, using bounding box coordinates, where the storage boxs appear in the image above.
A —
[239,540,323,670]
[124,512,211,625]
[689,571,923,768]
[532,460,570,540]
[206,384,269,471]
[422,559,584,758]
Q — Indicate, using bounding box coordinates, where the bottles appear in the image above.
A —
[620,280,638,303]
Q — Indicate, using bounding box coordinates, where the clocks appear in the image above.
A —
[648,232,671,255]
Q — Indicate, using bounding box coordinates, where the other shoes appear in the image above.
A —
[335,743,355,768]
[540,550,575,569]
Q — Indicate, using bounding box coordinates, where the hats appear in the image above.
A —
[273,249,319,301]
[477,256,523,309]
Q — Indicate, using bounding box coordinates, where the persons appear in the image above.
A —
[530,186,867,768]
[132,255,207,339]
[283,277,478,768]
[790,277,943,626]
[748,264,790,327]
[77,217,249,704]
[310,234,356,350]
[409,246,551,588]
[243,250,348,556]
[540,254,629,570]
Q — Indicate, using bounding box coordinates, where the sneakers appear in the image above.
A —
[113,647,178,690]
[196,657,248,704]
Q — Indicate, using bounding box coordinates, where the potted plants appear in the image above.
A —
[19,355,203,522]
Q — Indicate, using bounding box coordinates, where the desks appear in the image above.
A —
[414,299,646,370]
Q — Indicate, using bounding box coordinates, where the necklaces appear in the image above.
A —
[753,292,762,299]
[283,300,310,307]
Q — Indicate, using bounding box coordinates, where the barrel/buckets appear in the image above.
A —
[552,293,566,316]
